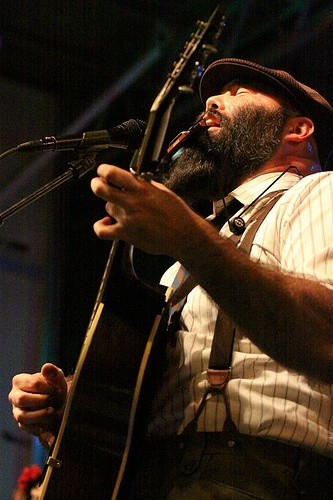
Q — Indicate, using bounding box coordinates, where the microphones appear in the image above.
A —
[17,119,148,154]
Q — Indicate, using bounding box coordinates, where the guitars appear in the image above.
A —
[38,3,230,499]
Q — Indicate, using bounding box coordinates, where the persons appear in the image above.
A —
[8,59,332,499]
